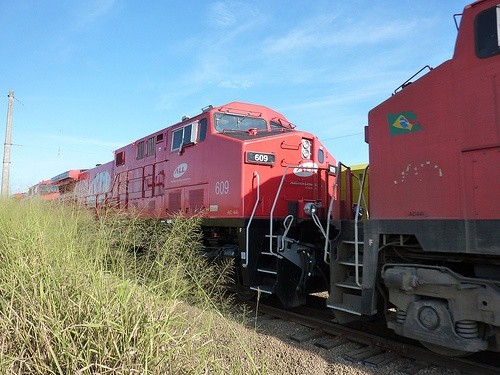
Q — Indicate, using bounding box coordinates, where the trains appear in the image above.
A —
[8,0,500,364]
